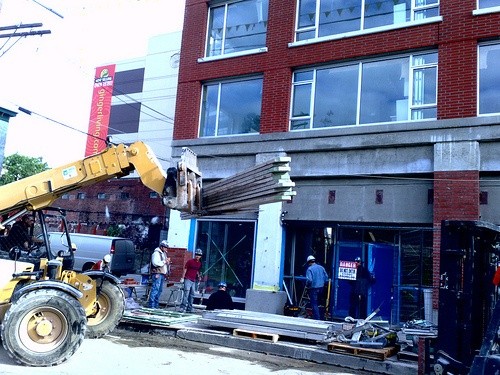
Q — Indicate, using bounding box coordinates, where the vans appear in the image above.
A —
[34,231,137,275]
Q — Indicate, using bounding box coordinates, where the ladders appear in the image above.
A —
[298,283,313,310]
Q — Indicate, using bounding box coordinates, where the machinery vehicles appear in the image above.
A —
[0,139,208,369]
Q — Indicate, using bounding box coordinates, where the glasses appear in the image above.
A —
[195,253,202,256]
[355,261,360,263]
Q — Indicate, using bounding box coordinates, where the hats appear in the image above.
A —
[307,255,315,262]
[218,282,227,287]
[355,257,361,261]
[104,255,113,263]
[71,243,78,249]
[195,249,202,255]
[159,241,169,248]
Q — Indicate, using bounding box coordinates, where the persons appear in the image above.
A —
[90,254,112,271]
[305,255,328,320]
[347,256,374,323]
[148,240,171,309]
[205,281,236,310]
[178,248,203,313]
[61,243,77,270]
